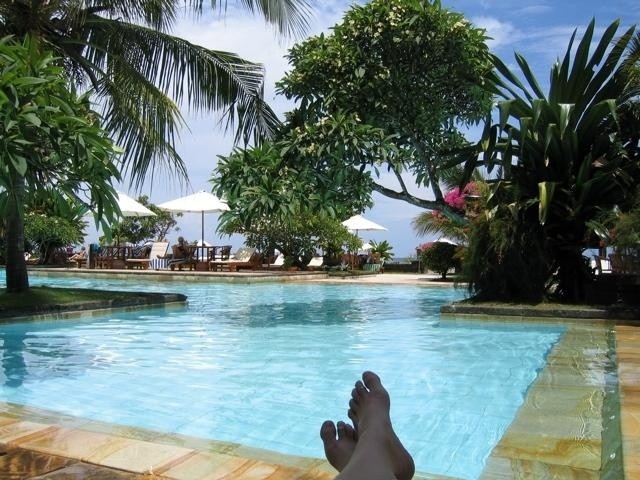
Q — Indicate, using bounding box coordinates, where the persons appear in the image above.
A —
[319,369,415,480]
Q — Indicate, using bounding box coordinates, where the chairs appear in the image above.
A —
[167,242,199,270]
[262,253,284,271]
[127,242,169,270]
[306,256,324,270]
[211,248,254,272]
[592,253,639,275]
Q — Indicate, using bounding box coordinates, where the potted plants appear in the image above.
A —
[283,255,298,271]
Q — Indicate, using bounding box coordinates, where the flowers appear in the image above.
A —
[412,180,490,241]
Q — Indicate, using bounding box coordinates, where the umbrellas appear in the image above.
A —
[157,190,244,263]
[340,213,387,235]
[85,187,158,259]
